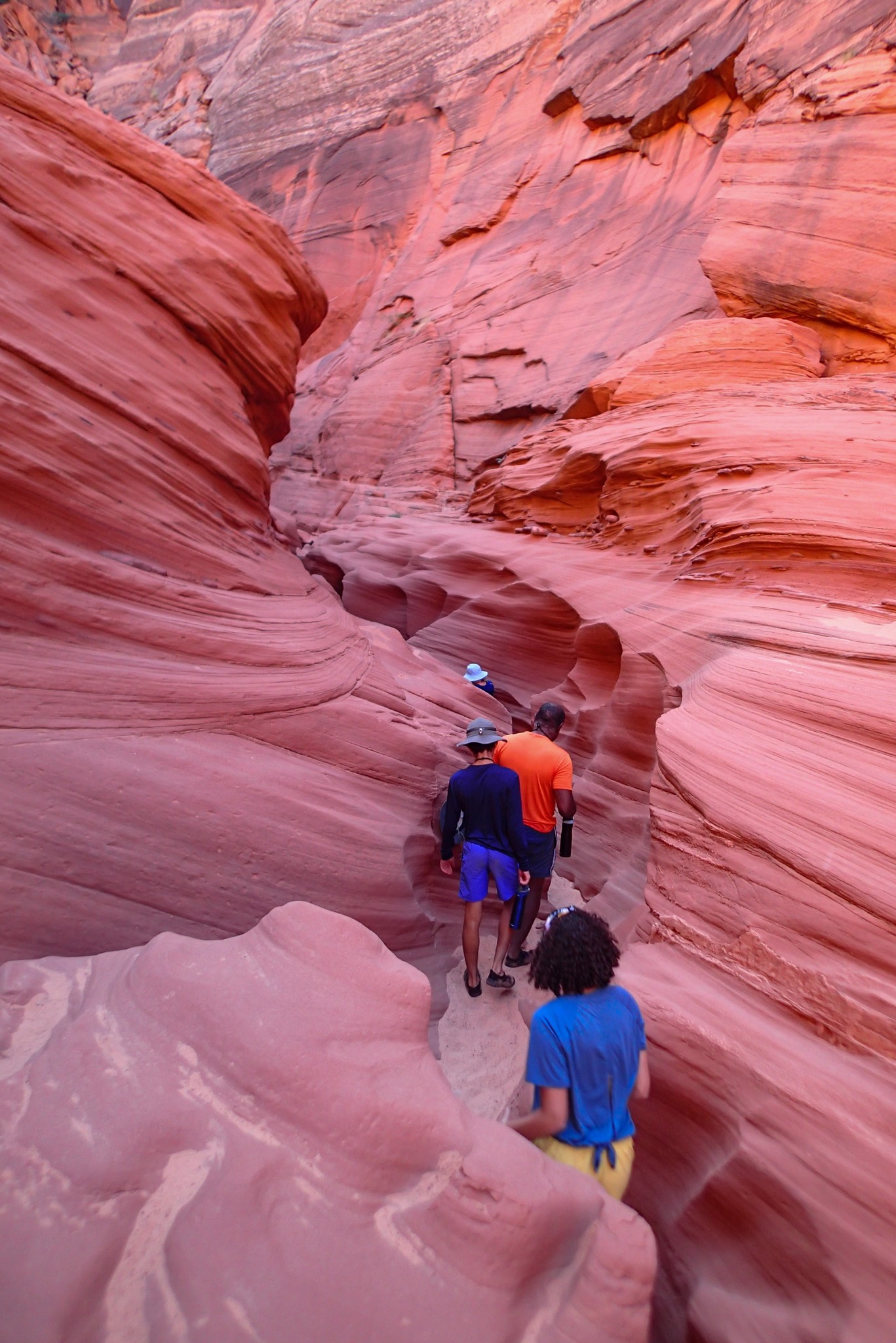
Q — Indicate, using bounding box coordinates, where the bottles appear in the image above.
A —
[509,883,530,929]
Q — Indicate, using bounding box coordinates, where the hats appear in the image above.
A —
[439,800,465,844]
[456,717,509,746]
[463,664,488,681]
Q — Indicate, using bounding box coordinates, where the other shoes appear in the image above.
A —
[486,969,515,988]
[504,950,537,967]
[463,968,482,998]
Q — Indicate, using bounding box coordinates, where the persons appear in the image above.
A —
[464,664,494,694]
[439,717,530,997]
[492,701,574,967]
[511,904,652,1205]
